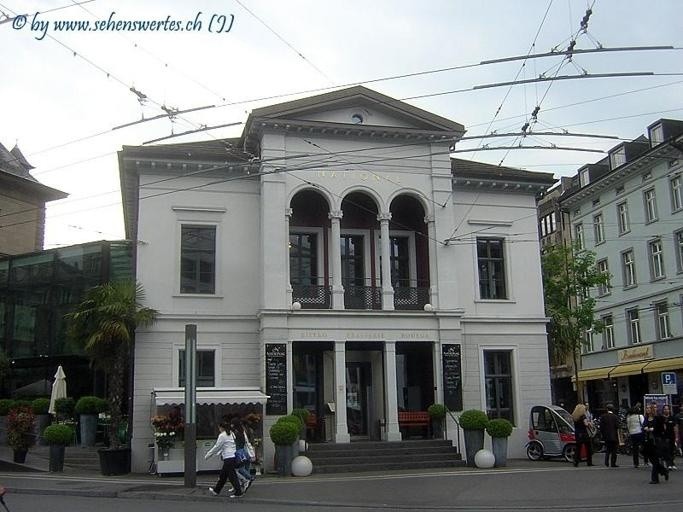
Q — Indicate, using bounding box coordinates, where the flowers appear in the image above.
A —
[150,413,177,450]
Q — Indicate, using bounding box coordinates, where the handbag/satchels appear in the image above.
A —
[235,449,251,467]
[244,433,256,462]
[587,424,598,438]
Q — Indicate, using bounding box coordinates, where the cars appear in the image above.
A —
[525,403,579,463]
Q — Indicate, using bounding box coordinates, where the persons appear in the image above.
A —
[557,398,683,484]
[205,418,256,498]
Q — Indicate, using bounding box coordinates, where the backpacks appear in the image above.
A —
[233,431,245,449]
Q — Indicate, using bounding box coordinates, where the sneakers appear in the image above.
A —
[636,465,641,468]
[228,476,255,499]
[207,487,218,496]
[645,464,649,468]
[668,466,678,471]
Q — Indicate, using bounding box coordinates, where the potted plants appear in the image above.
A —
[426,404,513,467]
[6,397,130,476]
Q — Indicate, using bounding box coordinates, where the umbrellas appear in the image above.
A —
[48,366,66,423]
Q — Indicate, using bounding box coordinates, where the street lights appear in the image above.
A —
[185,324,197,486]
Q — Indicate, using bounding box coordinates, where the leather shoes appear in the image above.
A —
[611,465,620,467]
[588,464,596,466]
[648,481,659,484]
[665,470,670,481]
[574,462,577,467]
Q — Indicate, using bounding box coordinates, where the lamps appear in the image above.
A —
[269,406,311,476]
